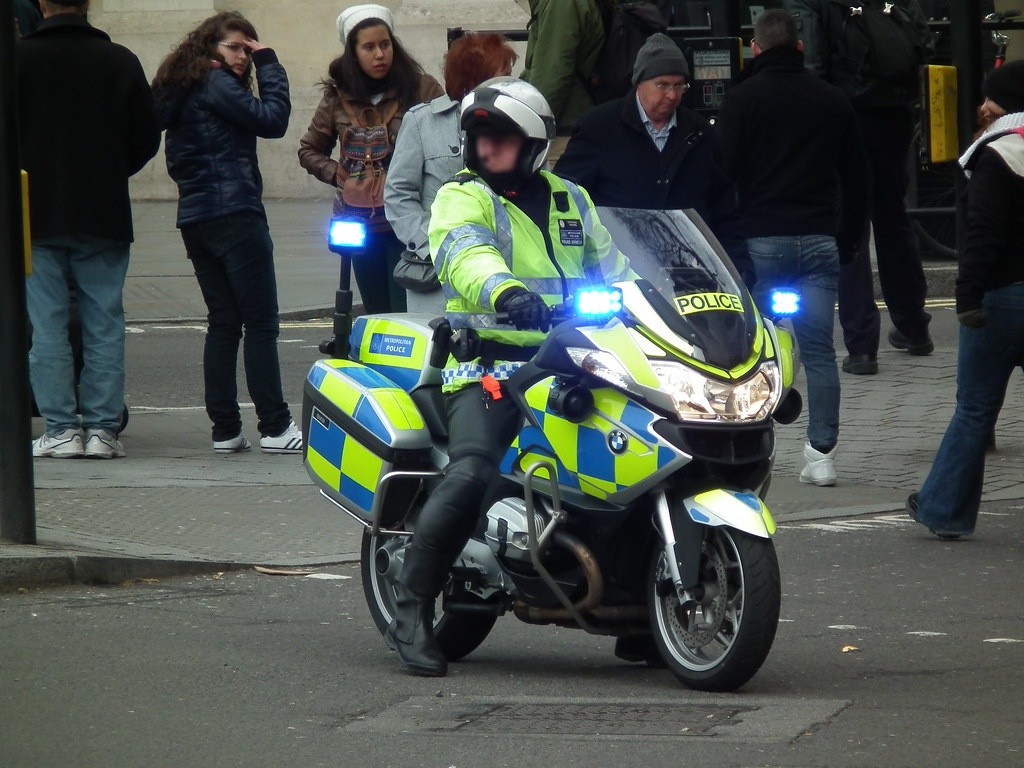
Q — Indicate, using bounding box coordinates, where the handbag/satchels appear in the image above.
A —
[393,249,444,294]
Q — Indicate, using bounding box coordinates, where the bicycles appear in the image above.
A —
[903,12,1024,261]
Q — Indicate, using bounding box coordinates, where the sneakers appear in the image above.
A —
[213,426,251,455]
[888,320,934,355]
[907,491,959,540]
[83,427,126,459]
[799,438,839,486]
[842,343,878,375]
[260,421,303,454]
[32,426,85,458]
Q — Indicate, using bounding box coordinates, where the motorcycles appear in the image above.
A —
[304,207,804,695]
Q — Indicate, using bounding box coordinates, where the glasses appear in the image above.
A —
[750,38,762,50]
[648,78,690,94]
[213,40,253,58]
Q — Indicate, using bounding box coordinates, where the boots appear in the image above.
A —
[385,546,454,676]
[614,632,671,669]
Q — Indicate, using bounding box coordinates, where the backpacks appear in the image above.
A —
[586,0,669,106]
[803,0,922,108]
[333,82,398,225]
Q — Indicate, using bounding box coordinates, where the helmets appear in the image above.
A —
[460,76,557,180]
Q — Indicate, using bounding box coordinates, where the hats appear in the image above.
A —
[337,4,394,48]
[631,34,689,89]
[984,59,1024,113]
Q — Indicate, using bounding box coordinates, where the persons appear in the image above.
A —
[906,60,1024,538]
[552,33,758,329]
[149,10,302,452]
[383,76,669,676]
[295,5,445,314]
[715,10,854,486]
[517,0,666,174]
[382,32,515,314]
[784,0,933,374]
[0,0,161,457]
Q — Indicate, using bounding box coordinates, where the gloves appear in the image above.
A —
[957,309,987,329]
[495,286,551,333]
